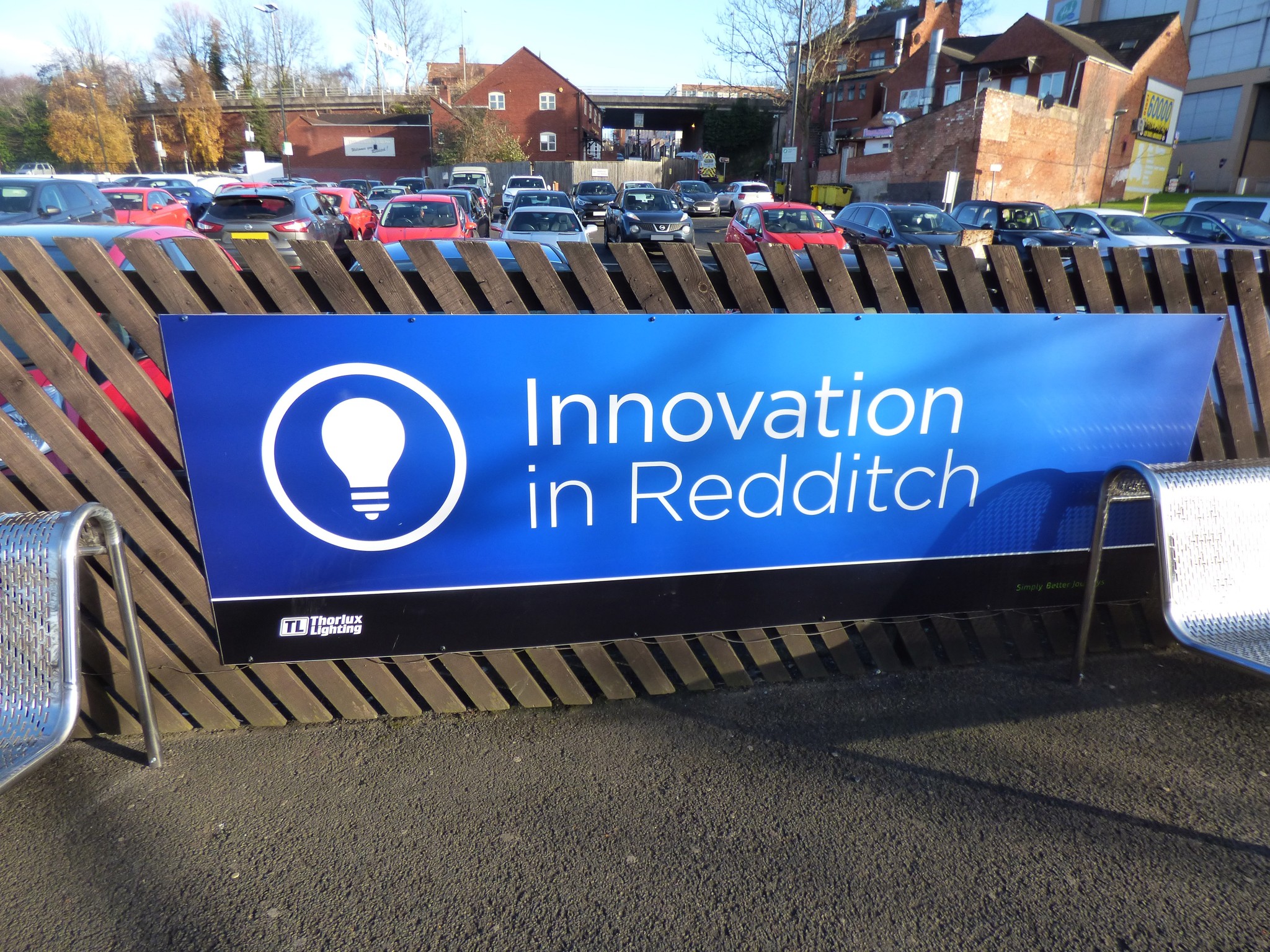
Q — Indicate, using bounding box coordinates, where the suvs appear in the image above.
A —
[941,200,1098,272]
[197,184,356,271]
[602,188,696,252]
[0,176,119,225]
[14,163,56,175]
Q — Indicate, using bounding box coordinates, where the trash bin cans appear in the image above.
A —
[817,183,828,207]
[825,183,854,209]
[1235,178,1247,195]
[809,184,818,206]
[774,179,786,194]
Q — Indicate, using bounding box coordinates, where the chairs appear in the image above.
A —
[600,185,608,194]
[0,502,165,795]
[652,196,662,210]
[627,195,641,208]
[1070,459,1270,681]
[522,197,532,204]
[551,198,560,206]
[549,213,572,231]
[432,205,454,226]
[1012,210,1027,229]
[1111,217,1123,232]
[394,205,422,226]
[513,179,520,186]
[475,178,485,187]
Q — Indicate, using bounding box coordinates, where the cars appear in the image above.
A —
[681,249,1002,313]
[725,201,852,255]
[617,153,624,161]
[957,195,1270,433]
[95,162,656,231]
[667,180,720,217]
[716,180,774,217]
[365,194,477,245]
[490,206,598,252]
[325,238,592,314]
[0,222,244,476]
[830,201,967,264]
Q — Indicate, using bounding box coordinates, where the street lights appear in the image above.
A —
[1098,109,1129,208]
[461,7,468,88]
[78,82,110,173]
[254,3,291,179]
[45,58,69,103]
[728,9,735,98]
[278,30,297,97]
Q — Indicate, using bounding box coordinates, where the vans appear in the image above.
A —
[675,151,718,181]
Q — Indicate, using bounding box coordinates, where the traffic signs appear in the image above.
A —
[719,157,730,163]
